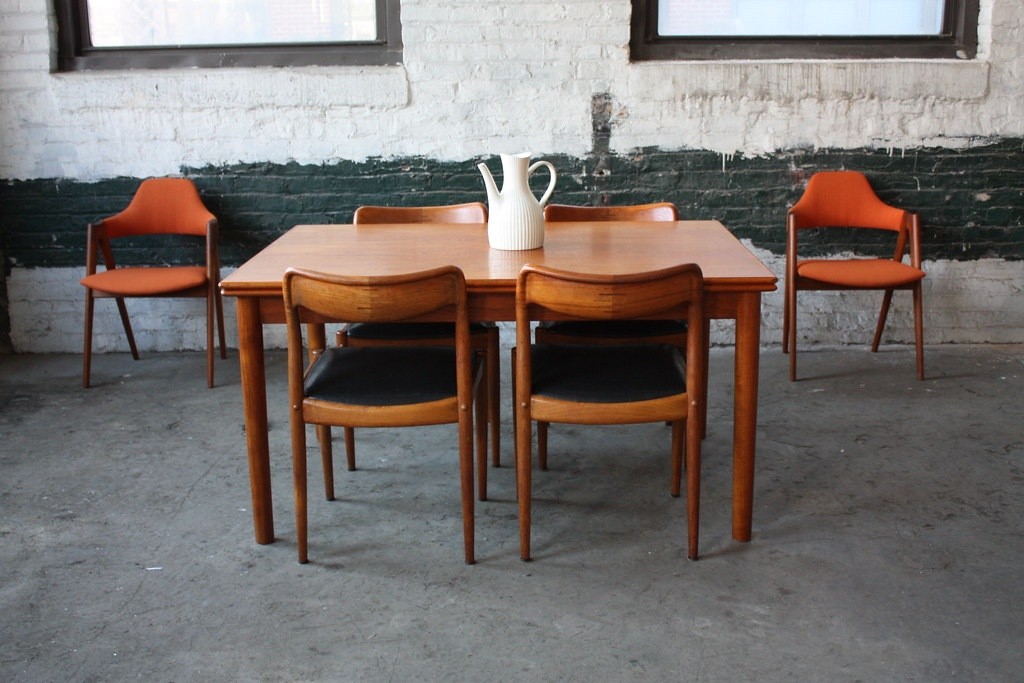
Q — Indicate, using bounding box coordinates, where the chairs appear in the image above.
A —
[80,178,227,389]
[534,204,690,471]
[512,264,705,563]
[782,170,926,382]
[281,266,488,565]
[335,201,501,472]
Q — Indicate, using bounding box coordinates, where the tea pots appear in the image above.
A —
[477,152,557,251]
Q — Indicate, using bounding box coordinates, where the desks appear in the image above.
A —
[217,219,778,545]
